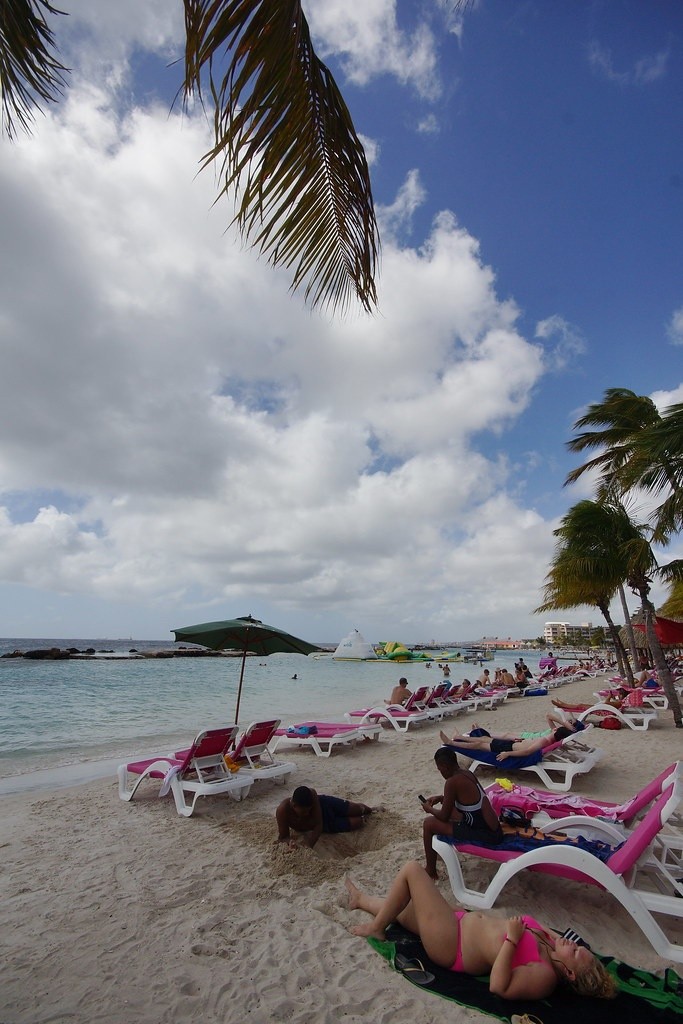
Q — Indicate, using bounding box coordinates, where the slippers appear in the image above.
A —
[395,954,435,985]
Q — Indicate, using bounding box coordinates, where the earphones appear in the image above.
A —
[568,970,572,975]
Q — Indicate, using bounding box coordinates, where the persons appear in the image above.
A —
[272,786,385,849]
[421,746,505,880]
[345,859,616,999]
[384,645,683,761]
[291,674,298,679]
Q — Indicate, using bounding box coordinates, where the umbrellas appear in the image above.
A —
[170,615,323,751]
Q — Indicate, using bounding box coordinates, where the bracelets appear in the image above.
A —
[506,937,517,948]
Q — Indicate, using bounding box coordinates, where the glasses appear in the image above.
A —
[405,683,408,684]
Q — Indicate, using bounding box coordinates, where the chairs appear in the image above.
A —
[431,780,683,963]
[363,686,445,721]
[444,685,472,715]
[167,719,297,800]
[344,704,429,733]
[455,680,484,712]
[462,723,603,762]
[527,660,683,690]
[116,726,255,818]
[240,728,361,758]
[288,721,383,745]
[489,683,542,696]
[593,686,678,710]
[488,688,508,701]
[553,689,660,730]
[441,731,596,792]
[473,692,504,709]
[483,759,683,869]
[424,683,462,718]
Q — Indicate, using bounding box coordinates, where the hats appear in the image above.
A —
[575,721,585,732]
[554,727,569,740]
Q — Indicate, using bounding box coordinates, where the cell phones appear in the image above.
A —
[418,795,426,803]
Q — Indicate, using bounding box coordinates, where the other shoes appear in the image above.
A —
[485,708,491,711]
[492,708,497,711]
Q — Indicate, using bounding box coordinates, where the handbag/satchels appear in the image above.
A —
[599,717,622,731]
[498,804,532,830]
